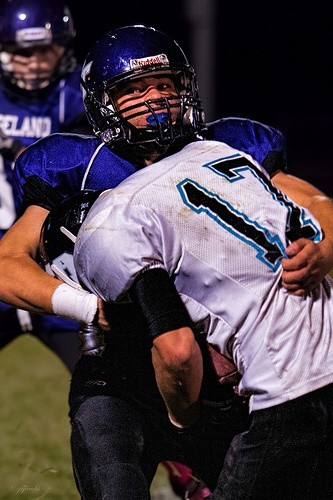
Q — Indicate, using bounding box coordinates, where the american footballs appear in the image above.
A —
[194,337,239,412]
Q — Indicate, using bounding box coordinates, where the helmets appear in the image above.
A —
[39,189,102,294]
[80,25,205,164]
[0,0,75,106]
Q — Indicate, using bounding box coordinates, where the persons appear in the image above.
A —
[0,0,333,500]
